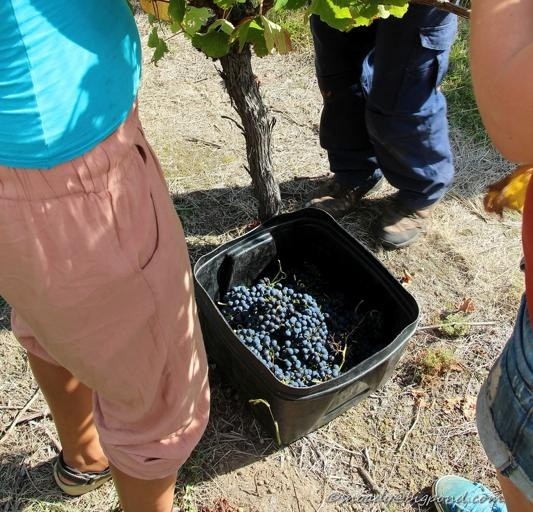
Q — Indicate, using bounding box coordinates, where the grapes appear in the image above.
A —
[218,258,384,390]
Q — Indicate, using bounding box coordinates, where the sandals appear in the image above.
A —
[52,450,113,496]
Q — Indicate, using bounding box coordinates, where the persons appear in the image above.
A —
[0,0,212,512]
[302,0,533,512]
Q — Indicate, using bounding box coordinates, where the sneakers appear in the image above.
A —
[302,175,384,216]
[427,473,506,512]
[378,186,441,249]
